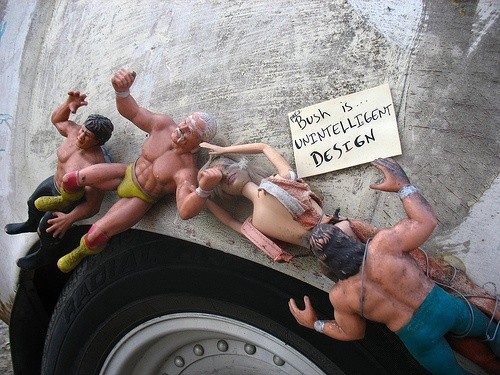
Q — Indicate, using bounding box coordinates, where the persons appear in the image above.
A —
[4,91,115,271]
[289,157,500,375]
[35,67,222,272]
[182,141,500,374]
